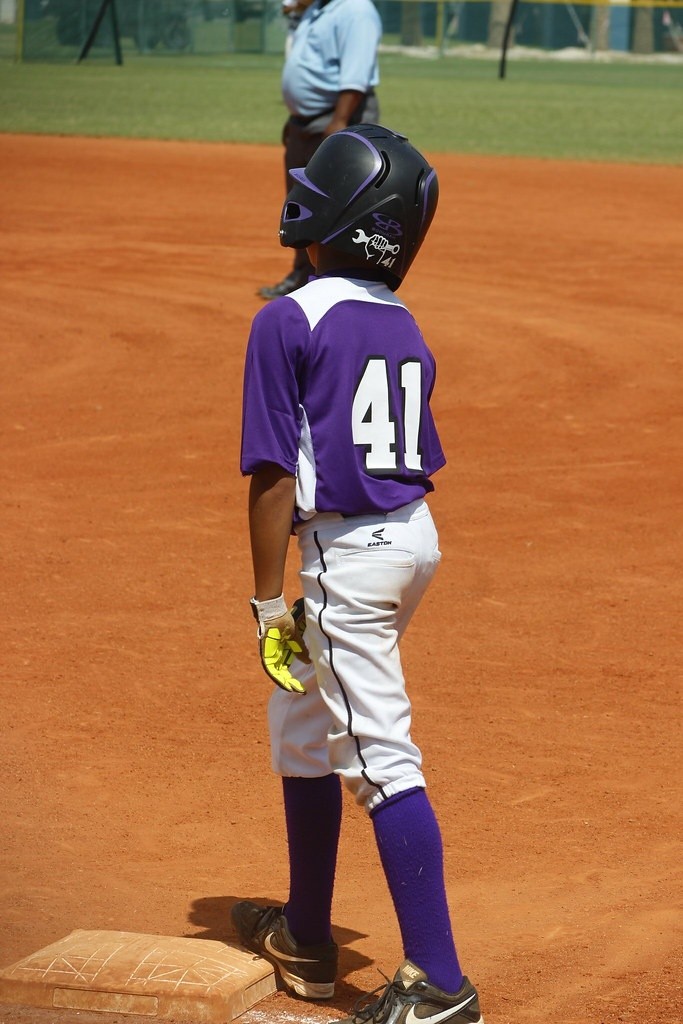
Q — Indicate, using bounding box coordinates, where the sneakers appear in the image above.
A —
[230,900,338,999]
[328,959,485,1024]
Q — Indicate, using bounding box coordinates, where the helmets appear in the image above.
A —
[277,122,440,294]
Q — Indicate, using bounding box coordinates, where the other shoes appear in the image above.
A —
[255,268,311,300]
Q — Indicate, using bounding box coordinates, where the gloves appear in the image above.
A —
[249,592,312,694]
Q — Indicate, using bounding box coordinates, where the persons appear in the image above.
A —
[231,124,482,1024]
[257,0,383,300]
